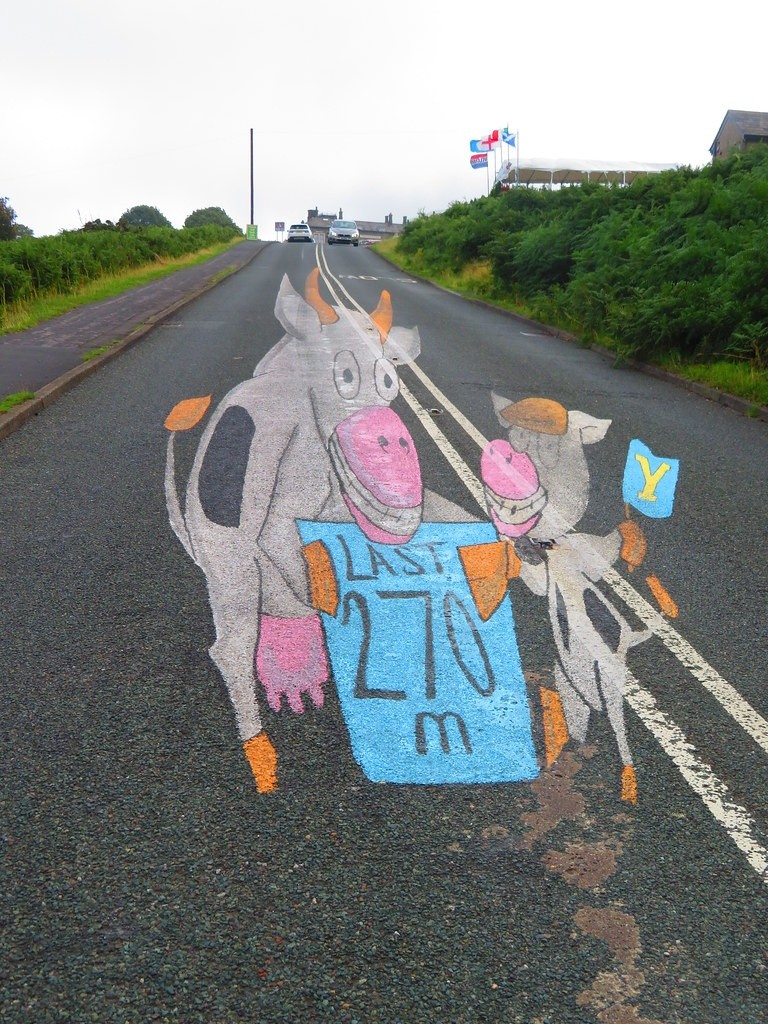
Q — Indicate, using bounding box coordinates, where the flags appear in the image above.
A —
[470,128,517,153]
[470,154,488,169]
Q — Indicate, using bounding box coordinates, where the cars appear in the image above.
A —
[328,219,359,246]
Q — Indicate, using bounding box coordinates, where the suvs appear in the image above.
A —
[288,224,313,243]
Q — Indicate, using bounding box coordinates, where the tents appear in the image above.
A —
[494,159,678,190]
